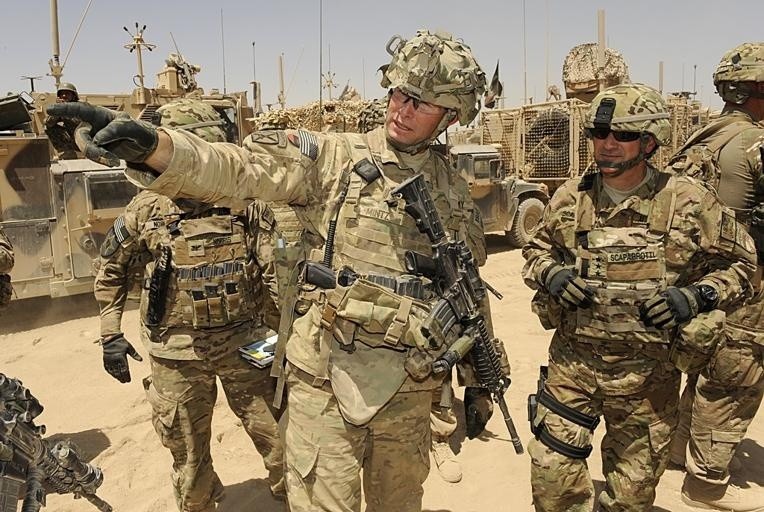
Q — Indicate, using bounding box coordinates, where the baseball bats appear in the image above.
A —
[391,173,527,454]
[305,264,354,355]
[0,374,116,512]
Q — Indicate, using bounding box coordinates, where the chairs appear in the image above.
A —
[672,434,742,471]
[431,433,462,483]
[681,471,764,511]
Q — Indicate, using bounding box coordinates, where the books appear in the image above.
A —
[238,333,280,368]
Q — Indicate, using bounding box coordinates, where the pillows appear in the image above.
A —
[57,83,80,100]
[581,83,673,148]
[151,98,234,143]
[714,43,764,84]
[377,30,487,126]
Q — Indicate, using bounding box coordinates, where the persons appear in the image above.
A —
[520,82,756,511]
[93,99,281,509]
[430,368,462,482]
[56,83,80,153]
[665,41,763,511]
[47,31,484,510]
[0,226,13,275]
[255,113,306,247]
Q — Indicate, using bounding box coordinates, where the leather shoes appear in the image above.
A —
[543,261,594,313]
[102,332,142,383]
[45,102,159,167]
[465,387,494,440]
[640,280,706,330]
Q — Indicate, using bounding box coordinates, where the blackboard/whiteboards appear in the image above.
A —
[446,90,702,251]
[0,0,266,314]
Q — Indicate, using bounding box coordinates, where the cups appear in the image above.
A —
[393,86,447,115]
[589,124,641,142]
